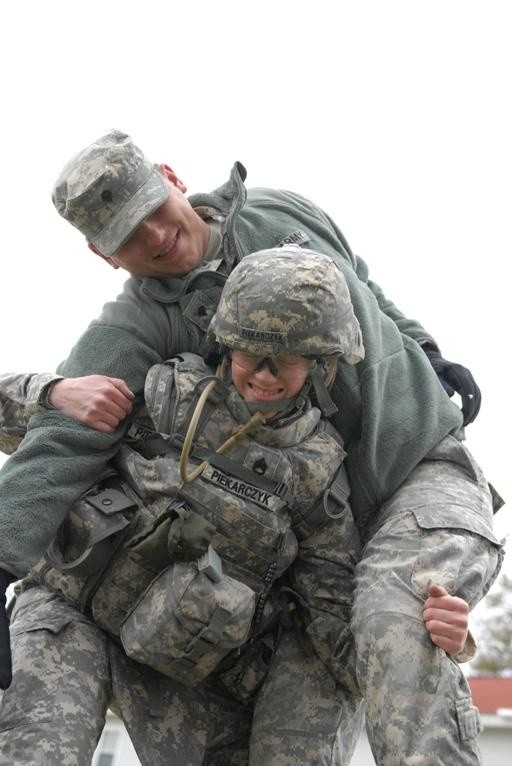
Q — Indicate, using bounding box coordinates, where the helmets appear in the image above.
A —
[205,244,366,367]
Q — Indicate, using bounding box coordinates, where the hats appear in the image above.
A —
[50,127,171,259]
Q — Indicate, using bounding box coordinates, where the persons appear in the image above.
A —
[2,239,481,766]
[1,129,506,766]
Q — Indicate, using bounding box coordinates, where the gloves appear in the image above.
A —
[427,347,481,427]
